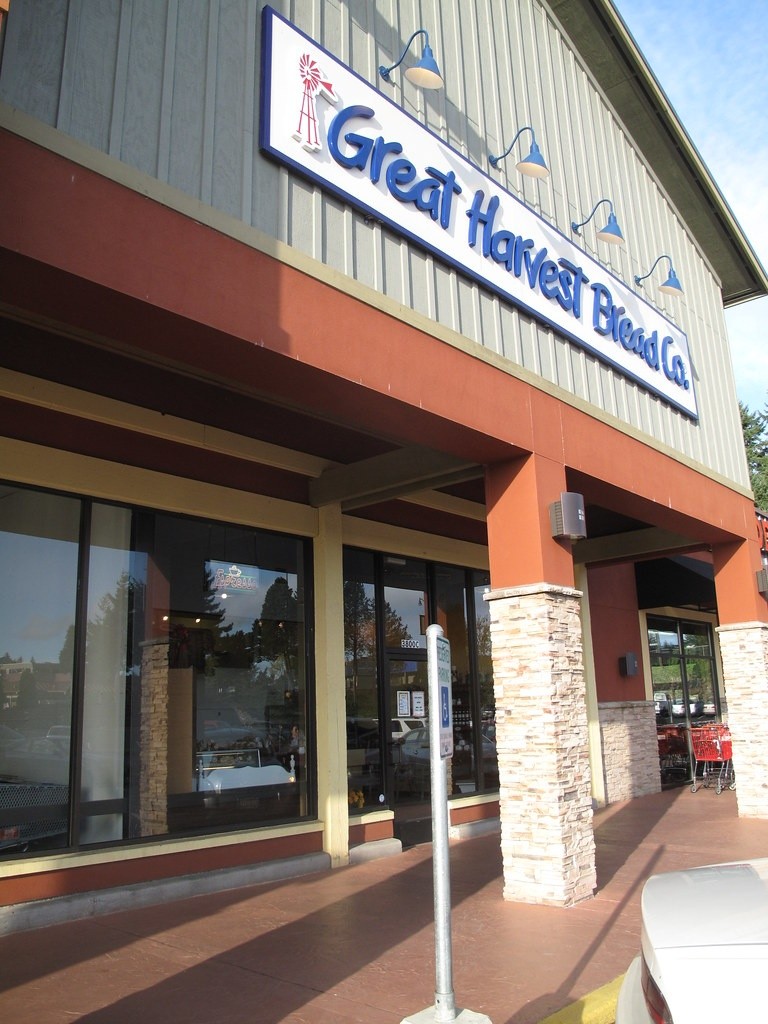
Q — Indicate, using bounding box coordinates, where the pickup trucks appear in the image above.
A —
[654,690,674,718]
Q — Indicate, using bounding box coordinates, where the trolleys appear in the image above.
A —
[690,721,738,795]
[658,723,704,786]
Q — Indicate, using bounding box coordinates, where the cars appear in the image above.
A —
[671,698,698,718]
[703,698,717,717]
[48,725,72,748]
[345,717,499,798]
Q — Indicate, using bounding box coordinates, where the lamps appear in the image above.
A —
[635,255,684,295]
[489,127,550,178]
[379,29,445,90]
[572,199,625,245]
